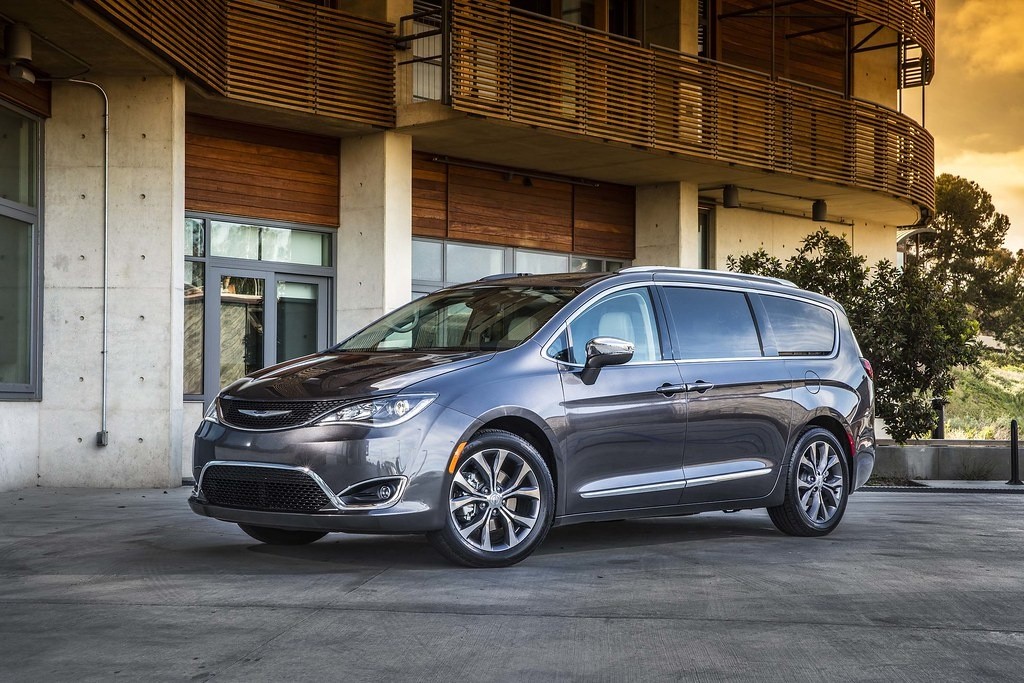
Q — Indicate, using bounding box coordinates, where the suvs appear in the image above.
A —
[185,264,875,569]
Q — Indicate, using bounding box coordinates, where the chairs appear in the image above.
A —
[598,312,641,365]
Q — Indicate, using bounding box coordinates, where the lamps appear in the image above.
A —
[812,200,827,221]
[723,184,740,209]
